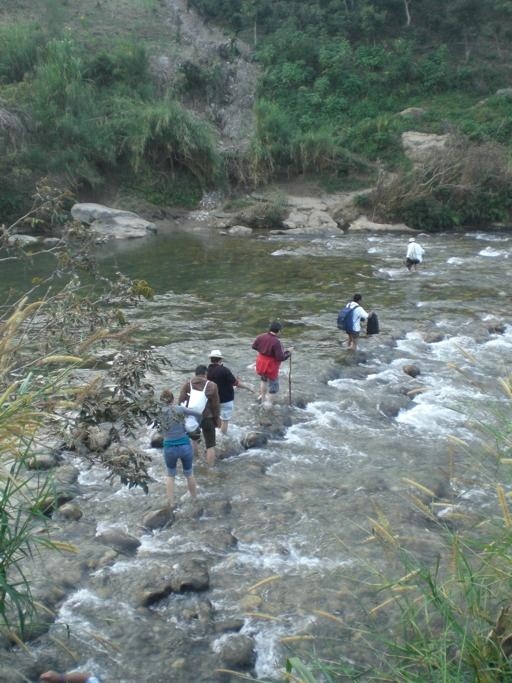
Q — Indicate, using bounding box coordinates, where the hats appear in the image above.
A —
[408,236,416,242]
[207,347,226,359]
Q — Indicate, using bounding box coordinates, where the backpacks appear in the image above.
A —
[185,376,212,415]
[335,301,361,331]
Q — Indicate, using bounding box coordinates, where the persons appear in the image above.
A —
[206,350,255,433]
[178,365,221,465]
[153,390,203,502]
[251,322,294,402]
[345,294,371,350]
[406,237,426,273]
